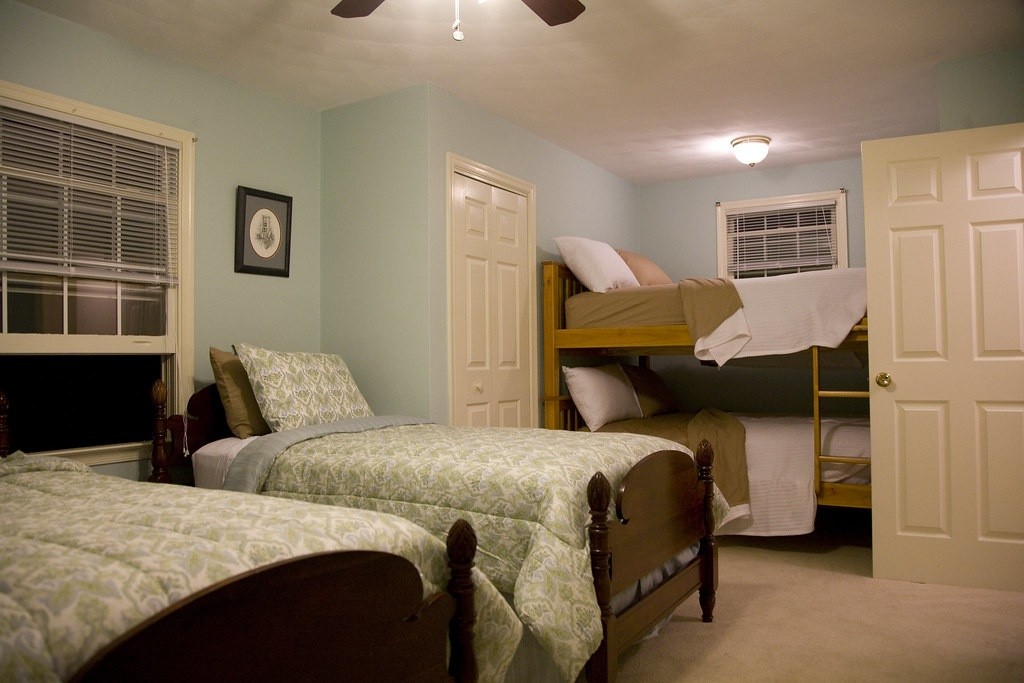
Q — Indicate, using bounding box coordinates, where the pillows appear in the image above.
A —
[232,341,377,434]
[624,365,684,419]
[613,247,673,288]
[562,363,643,432]
[208,346,271,438]
[554,235,639,294]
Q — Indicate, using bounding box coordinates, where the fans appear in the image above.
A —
[330,0,585,26]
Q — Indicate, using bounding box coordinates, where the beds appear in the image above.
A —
[541,258,872,511]
[0,446,524,683]
[151,379,716,683]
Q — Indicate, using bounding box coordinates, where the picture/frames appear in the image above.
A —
[235,185,294,277]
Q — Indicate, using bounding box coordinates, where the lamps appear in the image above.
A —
[731,135,771,167]
